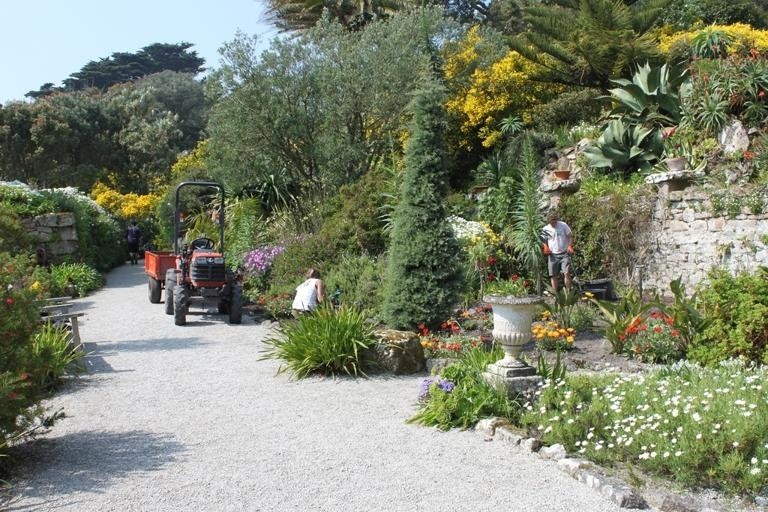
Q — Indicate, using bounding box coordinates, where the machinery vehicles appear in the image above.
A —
[145,181,242,326]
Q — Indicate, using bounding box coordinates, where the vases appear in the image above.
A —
[554,170,570,180]
[484,295,537,367]
[665,157,686,171]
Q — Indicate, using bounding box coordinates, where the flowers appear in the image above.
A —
[652,119,688,159]
[472,240,535,300]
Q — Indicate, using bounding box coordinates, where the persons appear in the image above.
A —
[290,268,326,320]
[123,221,142,264]
[540,211,575,291]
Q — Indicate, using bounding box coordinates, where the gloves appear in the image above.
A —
[567,244,574,254]
[543,244,550,256]
